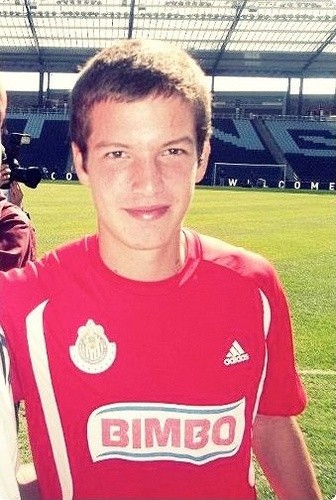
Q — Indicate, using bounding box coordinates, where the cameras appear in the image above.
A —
[0,133,42,189]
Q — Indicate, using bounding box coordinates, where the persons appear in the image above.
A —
[0,82,37,271]
[0,39,322,500]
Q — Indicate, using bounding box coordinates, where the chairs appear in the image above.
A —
[2,110,336,189]
